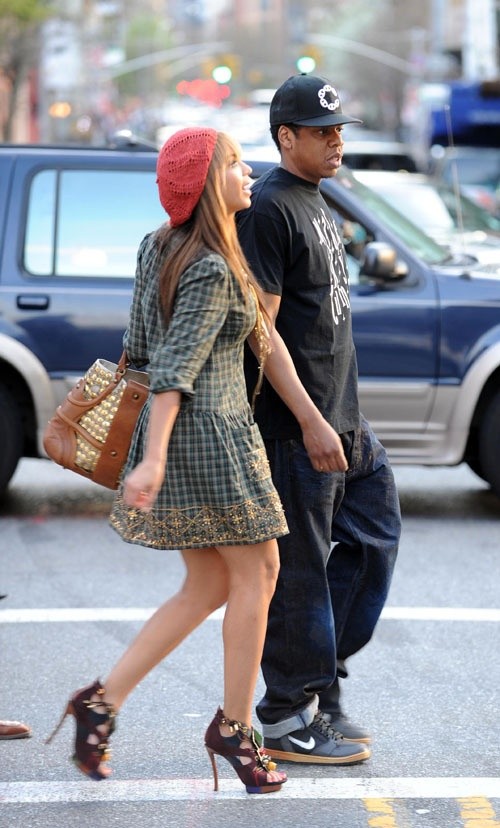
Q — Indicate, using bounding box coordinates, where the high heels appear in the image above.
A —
[203,706,288,793]
[44,677,120,780]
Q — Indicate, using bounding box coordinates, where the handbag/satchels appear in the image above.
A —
[43,358,151,490]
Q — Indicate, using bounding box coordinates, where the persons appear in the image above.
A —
[46,130,285,793]
[233,75,400,764]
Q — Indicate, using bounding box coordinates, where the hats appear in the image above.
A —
[157,127,217,228]
[269,71,363,127]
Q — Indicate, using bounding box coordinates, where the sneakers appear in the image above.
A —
[262,715,371,766]
[317,710,372,743]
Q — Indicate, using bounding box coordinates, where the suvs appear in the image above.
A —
[1,144,500,487]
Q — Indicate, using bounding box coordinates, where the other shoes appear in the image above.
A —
[0,719,30,740]
